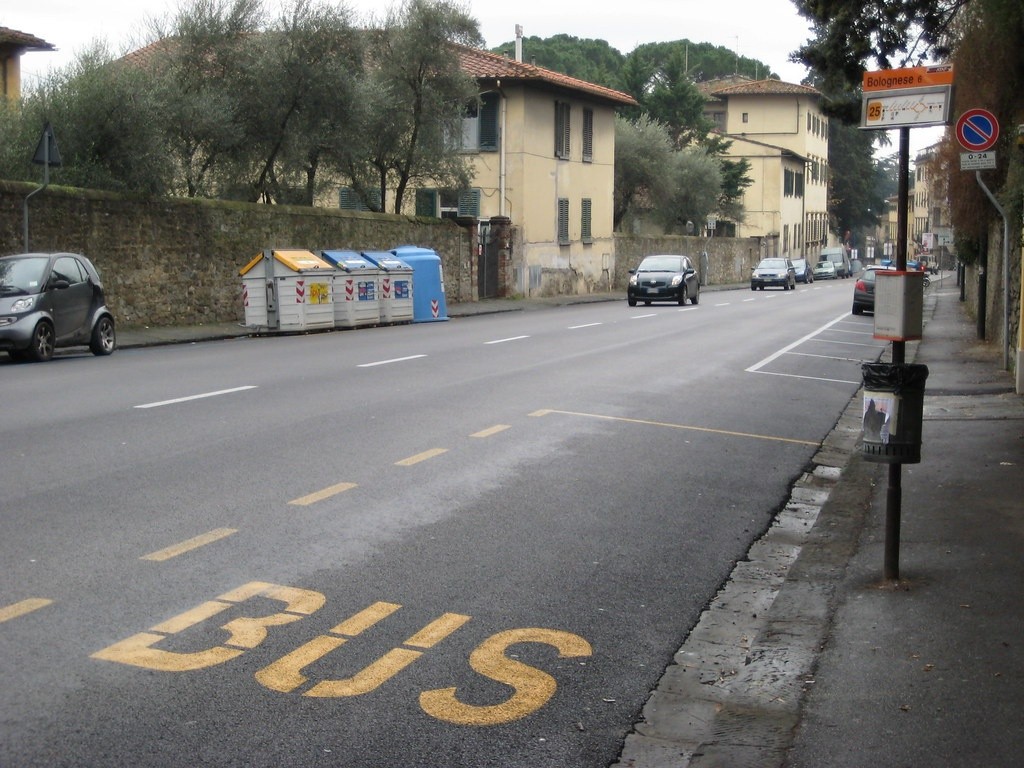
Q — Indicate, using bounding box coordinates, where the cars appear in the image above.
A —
[813,261,838,280]
[751,257,797,291]
[627,255,700,308]
[790,258,814,284]
[851,257,931,315]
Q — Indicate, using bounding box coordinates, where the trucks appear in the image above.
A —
[818,247,853,279]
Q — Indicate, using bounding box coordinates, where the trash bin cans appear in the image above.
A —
[360,250,415,326]
[313,249,381,331]
[860,362,929,464]
[389,246,451,323]
[237,247,336,338]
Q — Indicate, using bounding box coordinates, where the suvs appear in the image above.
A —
[915,252,940,275]
[0,251,117,362]
